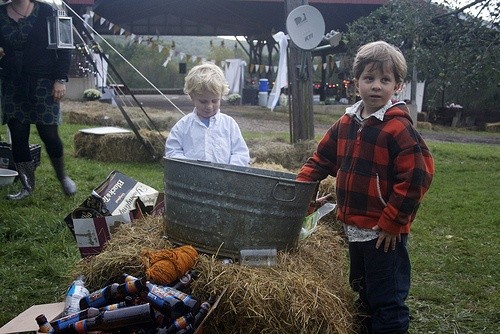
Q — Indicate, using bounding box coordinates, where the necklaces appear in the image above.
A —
[12,5,30,17]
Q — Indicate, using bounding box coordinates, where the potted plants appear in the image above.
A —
[83,89,101,101]
[228,93,240,106]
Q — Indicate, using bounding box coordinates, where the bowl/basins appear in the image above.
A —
[162,156,321,260]
[0,168,18,185]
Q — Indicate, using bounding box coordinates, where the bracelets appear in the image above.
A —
[57,79,64,84]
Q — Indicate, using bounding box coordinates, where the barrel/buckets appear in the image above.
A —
[259,79,268,92]
[258,92,268,106]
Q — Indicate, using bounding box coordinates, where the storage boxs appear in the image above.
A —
[64,170,164,257]
[0,285,229,334]
[0,141,41,172]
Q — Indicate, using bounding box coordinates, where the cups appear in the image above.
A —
[240,248,277,266]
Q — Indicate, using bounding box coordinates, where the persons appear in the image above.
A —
[0,0,76,199]
[297,40,434,334]
[165,63,250,166]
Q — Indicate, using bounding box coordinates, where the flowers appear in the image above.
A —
[280,94,287,106]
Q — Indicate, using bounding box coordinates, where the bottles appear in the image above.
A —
[35,272,211,334]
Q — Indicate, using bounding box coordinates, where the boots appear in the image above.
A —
[6,159,35,198]
[49,154,77,195]
